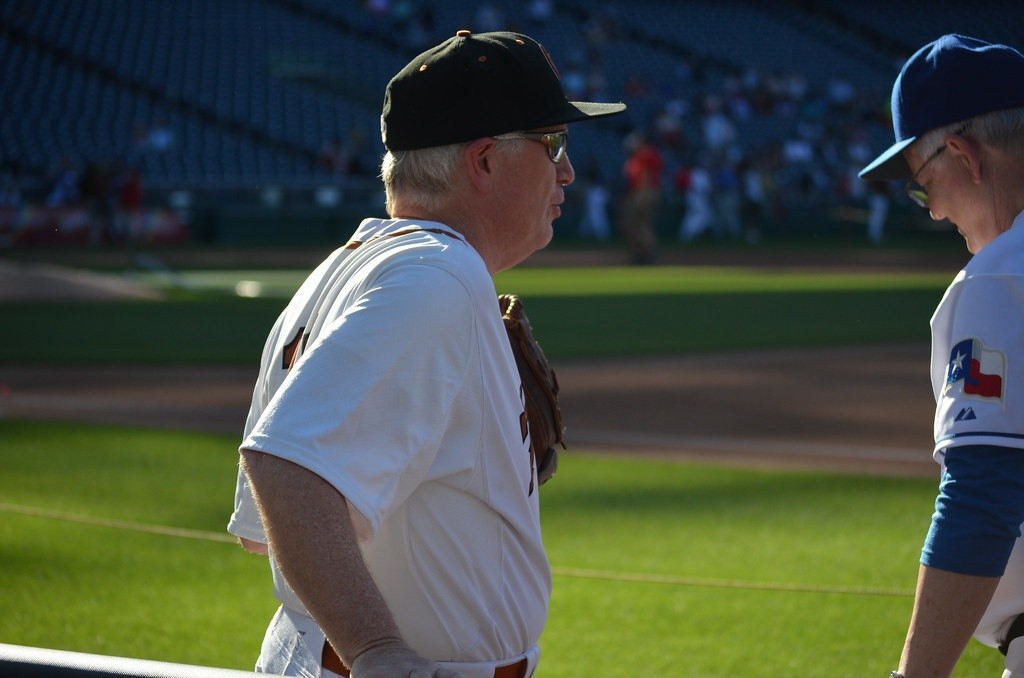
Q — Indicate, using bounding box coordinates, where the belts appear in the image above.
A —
[322,641,527,678]
[998,613,1024,655]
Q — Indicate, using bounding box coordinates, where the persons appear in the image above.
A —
[12,53,898,247]
[858,35,1023,678]
[223,29,574,676]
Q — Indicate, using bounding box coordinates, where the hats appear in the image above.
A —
[381,29,628,151]
[857,33,1024,178]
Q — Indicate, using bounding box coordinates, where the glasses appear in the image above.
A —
[492,131,568,163]
[906,123,969,208]
